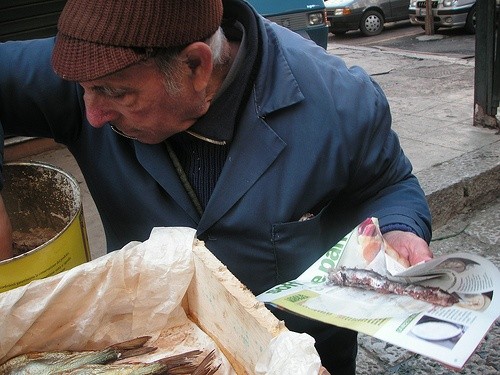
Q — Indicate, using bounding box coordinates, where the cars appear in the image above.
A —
[409,0,500,33]
[324,0,410,36]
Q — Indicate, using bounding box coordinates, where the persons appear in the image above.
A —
[1,1,433,374]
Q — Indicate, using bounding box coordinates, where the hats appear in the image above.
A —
[53,0,223,81]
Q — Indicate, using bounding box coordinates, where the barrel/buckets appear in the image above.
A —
[0,161,93,304]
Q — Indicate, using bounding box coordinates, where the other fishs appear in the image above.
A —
[328,266,462,308]
[0,336,222,375]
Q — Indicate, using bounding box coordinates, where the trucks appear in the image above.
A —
[248,0,328,51]
